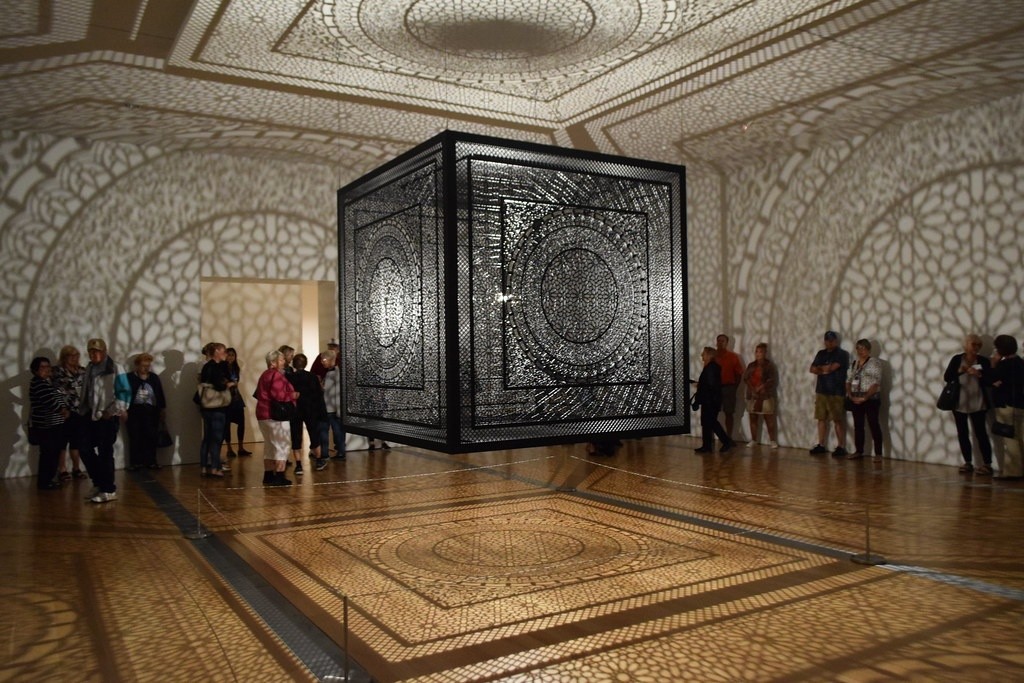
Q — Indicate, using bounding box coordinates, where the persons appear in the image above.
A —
[944,335,992,476]
[693,347,735,453]
[278,342,346,474]
[743,342,778,448]
[48,345,87,479]
[78,338,132,501]
[985,334,1024,478]
[846,339,883,462]
[29,356,73,490]
[192,342,237,479]
[367,437,391,450]
[225,348,252,456]
[715,334,742,436]
[125,353,173,469]
[256,349,300,484]
[809,330,850,456]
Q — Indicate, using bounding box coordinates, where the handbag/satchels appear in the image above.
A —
[269,398,298,421]
[201,387,231,407]
[937,381,959,410]
[27,426,45,444]
[991,420,1014,438]
[844,396,852,410]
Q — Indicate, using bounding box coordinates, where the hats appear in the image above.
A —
[825,331,837,341]
[327,337,339,345]
[87,338,106,351]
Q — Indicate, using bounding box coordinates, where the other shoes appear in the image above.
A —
[294,466,303,474]
[976,465,993,474]
[36,469,89,488]
[695,446,714,452]
[309,452,346,462]
[832,446,846,456]
[873,454,882,462]
[747,439,759,447]
[848,451,864,459]
[809,443,826,454]
[316,460,327,470]
[770,440,778,448]
[959,462,972,471]
[200,448,292,487]
[721,440,734,453]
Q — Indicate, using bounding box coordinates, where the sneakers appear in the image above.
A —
[91,491,117,503]
